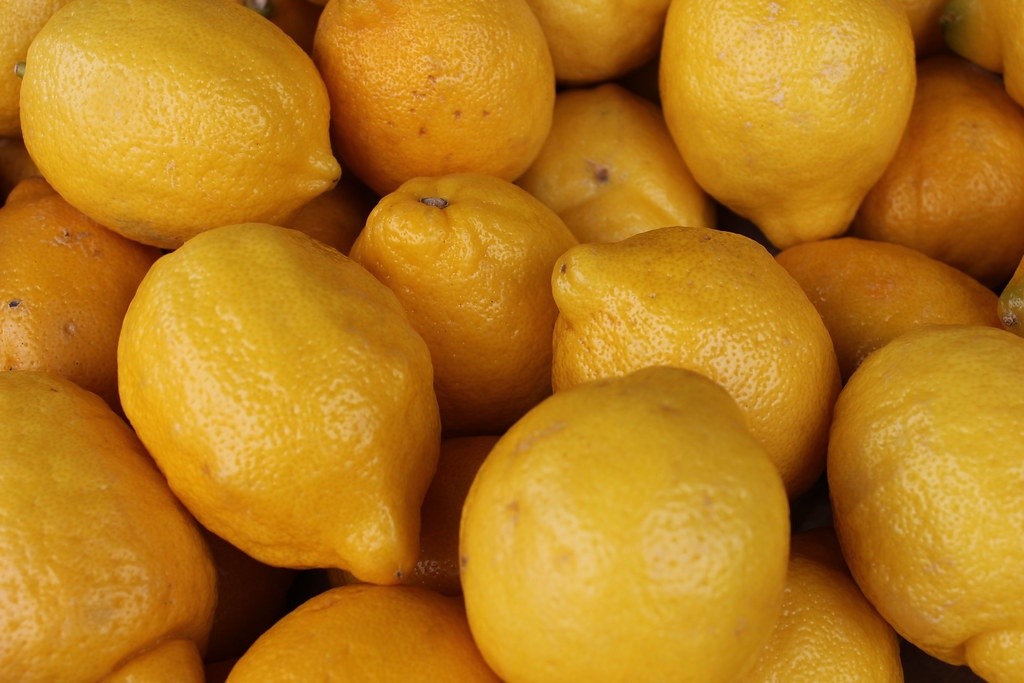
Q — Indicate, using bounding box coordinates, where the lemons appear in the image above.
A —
[0,0,1024,683]
